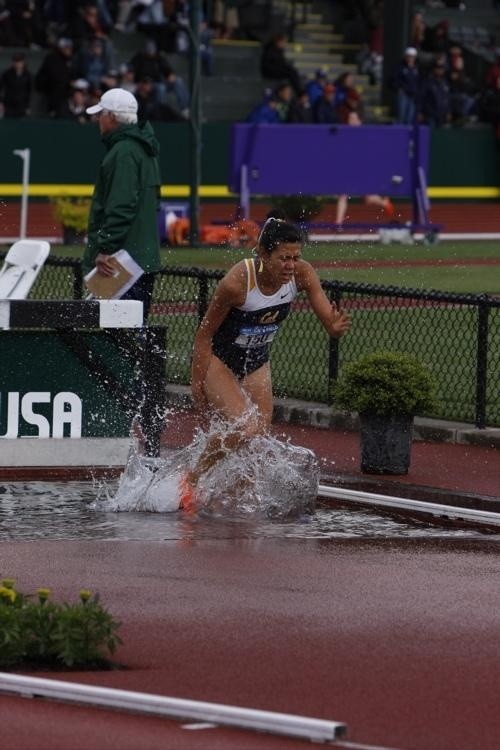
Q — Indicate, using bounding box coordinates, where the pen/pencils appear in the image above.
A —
[85,293,94,301]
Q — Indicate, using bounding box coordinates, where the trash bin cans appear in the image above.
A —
[202,49,218,78]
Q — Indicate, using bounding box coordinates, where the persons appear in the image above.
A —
[85,86,167,324]
[0,0,500,123]
[179,217,354,516]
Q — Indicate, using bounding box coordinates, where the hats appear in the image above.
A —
[85,88,139,116]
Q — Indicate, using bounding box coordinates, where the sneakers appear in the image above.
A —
[179,468,195,514]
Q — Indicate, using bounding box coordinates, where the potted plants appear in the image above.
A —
[326,347,443,475]
[54,197,93,245]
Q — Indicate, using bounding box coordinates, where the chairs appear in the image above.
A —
[0,239,51,328]
